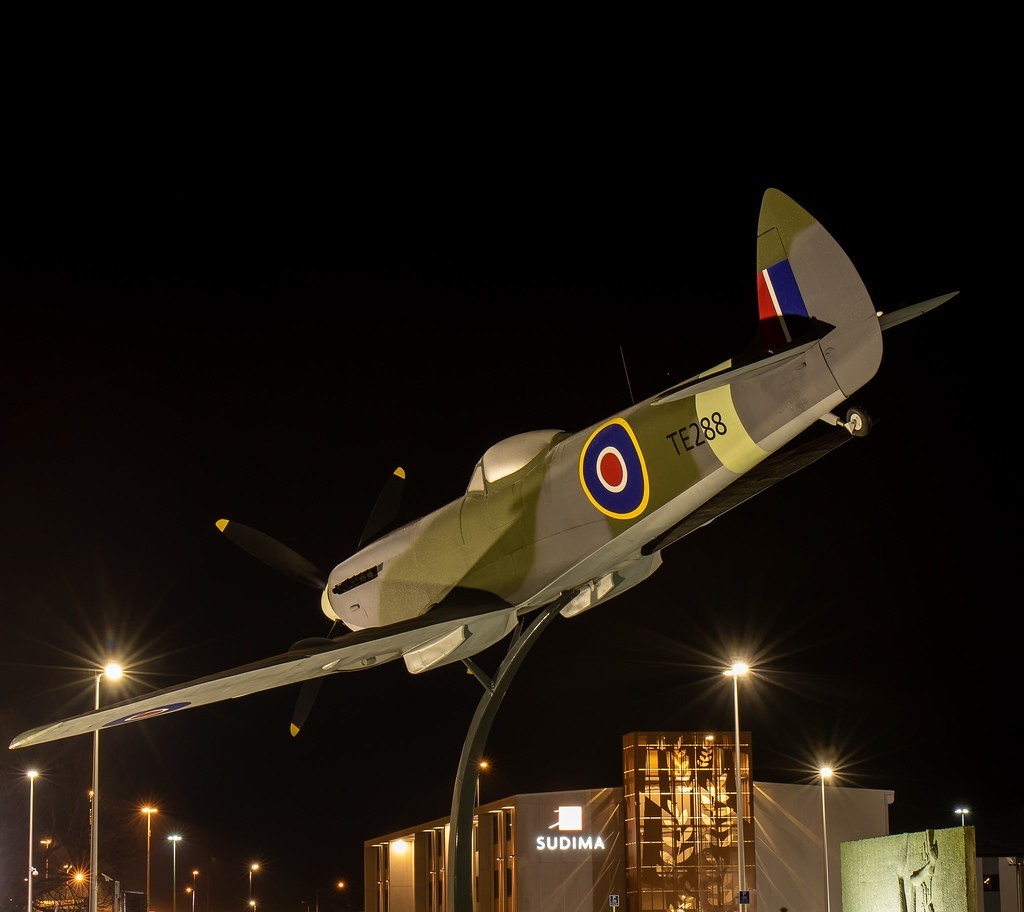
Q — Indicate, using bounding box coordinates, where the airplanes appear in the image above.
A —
[8,187,961,750]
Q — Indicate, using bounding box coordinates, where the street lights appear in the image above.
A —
[140,806,261,912]
[819,766,834,912]
[731,660,750,912]
[954,808,969,826]
[476,760,490,807]
[27,770,39,912]
[90,659,124,912]
